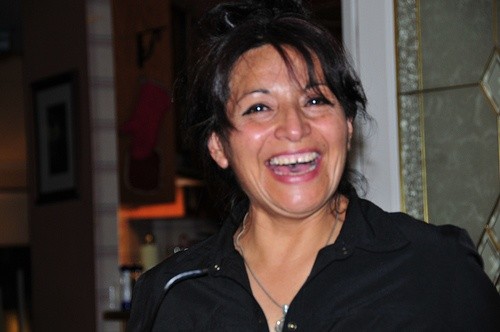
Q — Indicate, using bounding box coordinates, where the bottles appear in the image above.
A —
[141,233,159,269]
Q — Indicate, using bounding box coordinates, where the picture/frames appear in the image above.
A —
[29,67,81,207]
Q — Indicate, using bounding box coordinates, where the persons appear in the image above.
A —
[128,3,499,332]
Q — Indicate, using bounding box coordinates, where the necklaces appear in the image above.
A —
[236,194,342,332]
